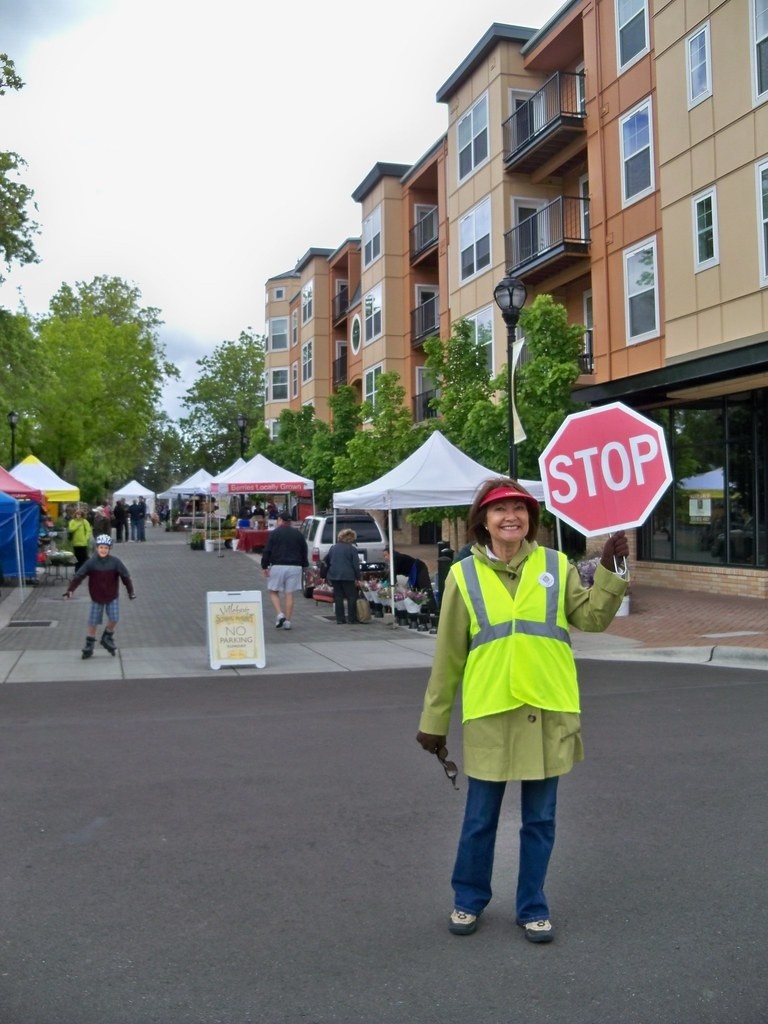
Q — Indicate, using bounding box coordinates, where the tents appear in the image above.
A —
[158,454,316,557]
[112,480,157,522]
[333,430,563,627]
[0,455,81,605]
[680,467,742,500]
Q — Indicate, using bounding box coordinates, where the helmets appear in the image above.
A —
[96,534,113,546]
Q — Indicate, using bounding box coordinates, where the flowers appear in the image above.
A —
[359,578,430,614]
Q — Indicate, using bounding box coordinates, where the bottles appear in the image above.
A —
[254,520,258,530]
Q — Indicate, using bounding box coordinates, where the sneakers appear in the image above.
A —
[448,909,484,935]
[523,919,554,943]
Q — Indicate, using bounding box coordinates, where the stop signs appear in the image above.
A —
[538,402,673,539]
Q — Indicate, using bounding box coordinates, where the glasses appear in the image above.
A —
[434,745,460,790]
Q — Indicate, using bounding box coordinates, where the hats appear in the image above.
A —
[479,487,537,507]
[275,511,292,521]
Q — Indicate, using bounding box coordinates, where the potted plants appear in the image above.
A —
[165,523,177,532]
[190,532,204,550]
[171,509,178,520]
[53,516,65,531]
[196,519,235,530]
[48,542,91,566]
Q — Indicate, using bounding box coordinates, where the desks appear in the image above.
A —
[236,526,273,554]
[313,587,336,607]
[36,559,77,585]
[176,516,216,529]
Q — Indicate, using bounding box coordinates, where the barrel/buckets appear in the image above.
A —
[615,596,629,616]
[147,521,152,527]
[233,540,239,551]
[205,540,215,552]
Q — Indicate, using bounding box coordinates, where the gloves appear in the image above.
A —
[600,530,629,572]
[416,732,446,754]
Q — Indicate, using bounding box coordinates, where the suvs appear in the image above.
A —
[300,508,392,598]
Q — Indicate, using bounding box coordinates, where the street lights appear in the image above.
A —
[7,410,20,469]
[237,413,249,458]
[494,270,530,484]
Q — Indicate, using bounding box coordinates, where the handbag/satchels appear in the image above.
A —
[356,587,373,624]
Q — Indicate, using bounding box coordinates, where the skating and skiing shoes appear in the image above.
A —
[100,626,117,657]
[81,636,97,659]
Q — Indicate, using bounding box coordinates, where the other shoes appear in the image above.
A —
[275,614,286,628]
[284,621,291,629]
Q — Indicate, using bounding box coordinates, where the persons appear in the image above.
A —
[416,477,631,944]
[261,510,311,630]
[254,501,265,518]
[381,548,436,614]
[238,501,252,520]
[319,528,364,624]
[223,515,235,547]
[65,496,169,574]
[63,534,136,661]
